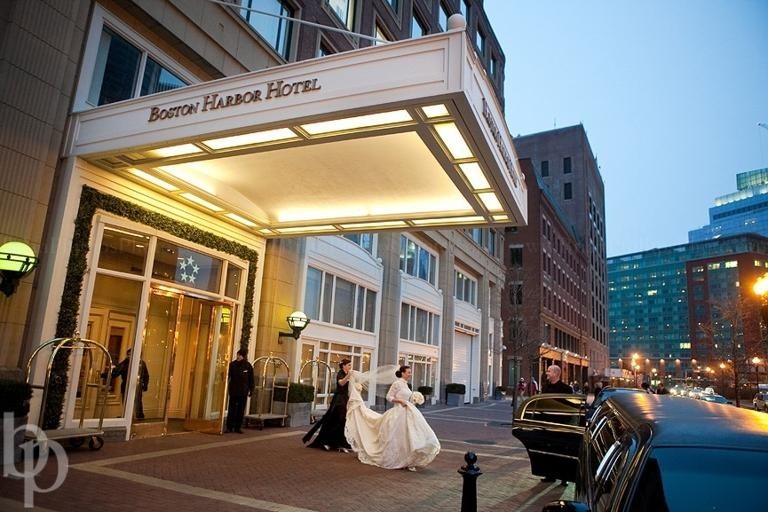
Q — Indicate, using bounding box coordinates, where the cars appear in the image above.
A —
[512,383,768,511]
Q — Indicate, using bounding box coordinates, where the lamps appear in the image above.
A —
[0,240,40,297]
[278,311,310,340]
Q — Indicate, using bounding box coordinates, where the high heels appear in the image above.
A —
[324,445,329,450]
[339,447,348,453]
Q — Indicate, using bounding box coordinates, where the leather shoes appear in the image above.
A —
[409,467,416,471]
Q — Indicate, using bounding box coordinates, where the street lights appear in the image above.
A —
[720,363,726,396]
[631,352,640,388]
[706,367,715,386]
[752,355,760,393]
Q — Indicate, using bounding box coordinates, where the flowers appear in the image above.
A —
[409,391,425,406]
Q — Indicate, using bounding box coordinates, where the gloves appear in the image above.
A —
[249,391,254,397]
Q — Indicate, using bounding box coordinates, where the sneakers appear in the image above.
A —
[224,429,232,433]
[234,428,244,433]
[562,481,568,486]
[541,478,555,482]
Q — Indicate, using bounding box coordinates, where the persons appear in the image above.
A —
[101,348,149,420]
[302,359,355,452]
[224,349,255,434]
[569,380,603,398]
[540,365,574,486]
[344,366,441,472]
[653,383,670,395]
[527,376,538,397]
[517,377,527,402]
